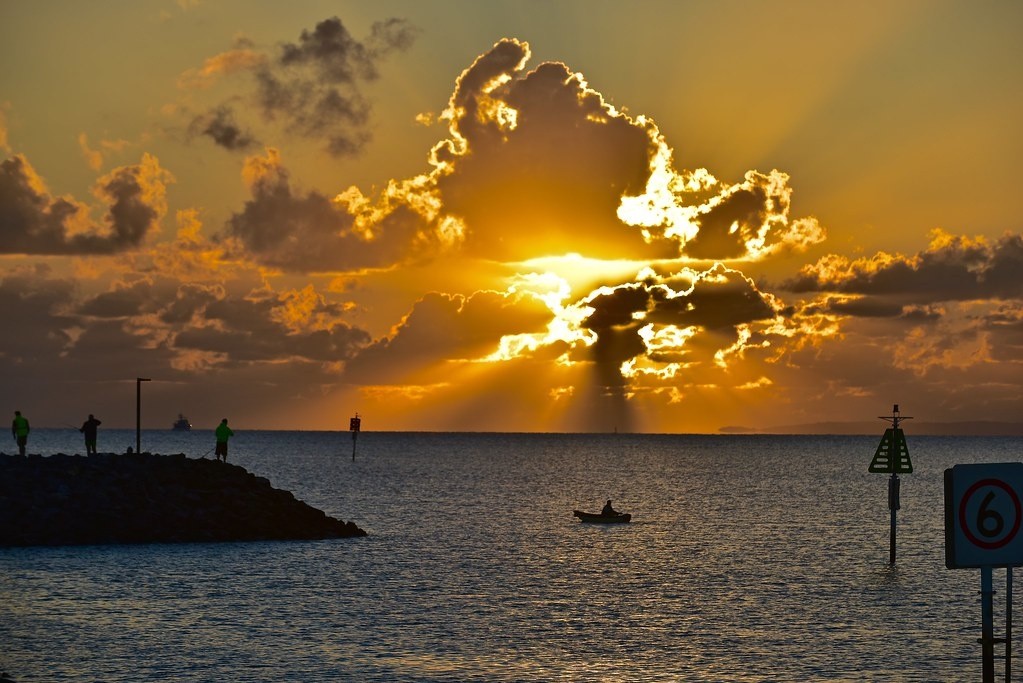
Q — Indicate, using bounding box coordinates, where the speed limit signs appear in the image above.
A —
[950,463,1023,569]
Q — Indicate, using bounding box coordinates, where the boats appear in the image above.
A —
[573,510,633,524]
[172,414,192,434]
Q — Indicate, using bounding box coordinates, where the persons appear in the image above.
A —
[80,414,101,456]
[602,500,617,515]
[12,411,30,456]
[215,419,234,463]
